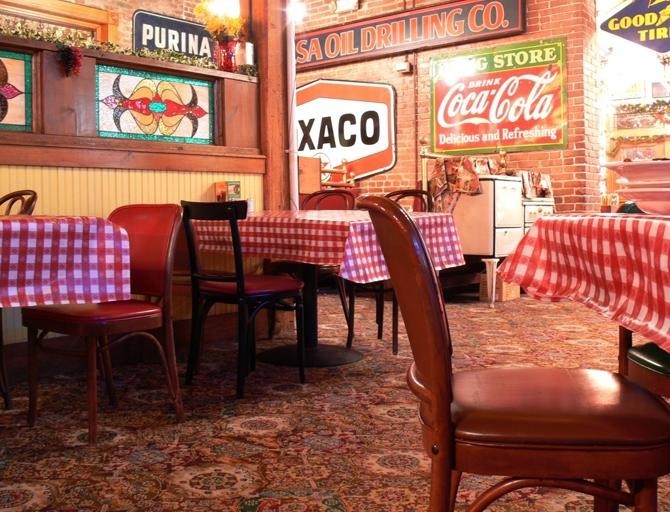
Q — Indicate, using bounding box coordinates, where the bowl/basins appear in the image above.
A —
[601,160,670,216]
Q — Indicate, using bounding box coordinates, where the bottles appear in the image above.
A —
[497,147,509,175]
[247,197,256,213]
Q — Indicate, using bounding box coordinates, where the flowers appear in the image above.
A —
[194,0,245,41]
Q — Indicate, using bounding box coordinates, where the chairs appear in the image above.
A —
[344,187,454,357]
[262,187,357,340]
[21,201,185,446]
[180,199,309,400]
[355,193,669,512]
[0,189,38,411]
[615,200,670,402]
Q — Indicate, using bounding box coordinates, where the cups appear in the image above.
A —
[600,205,611,213]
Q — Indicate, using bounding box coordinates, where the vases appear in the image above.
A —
[214,37,239,73]
